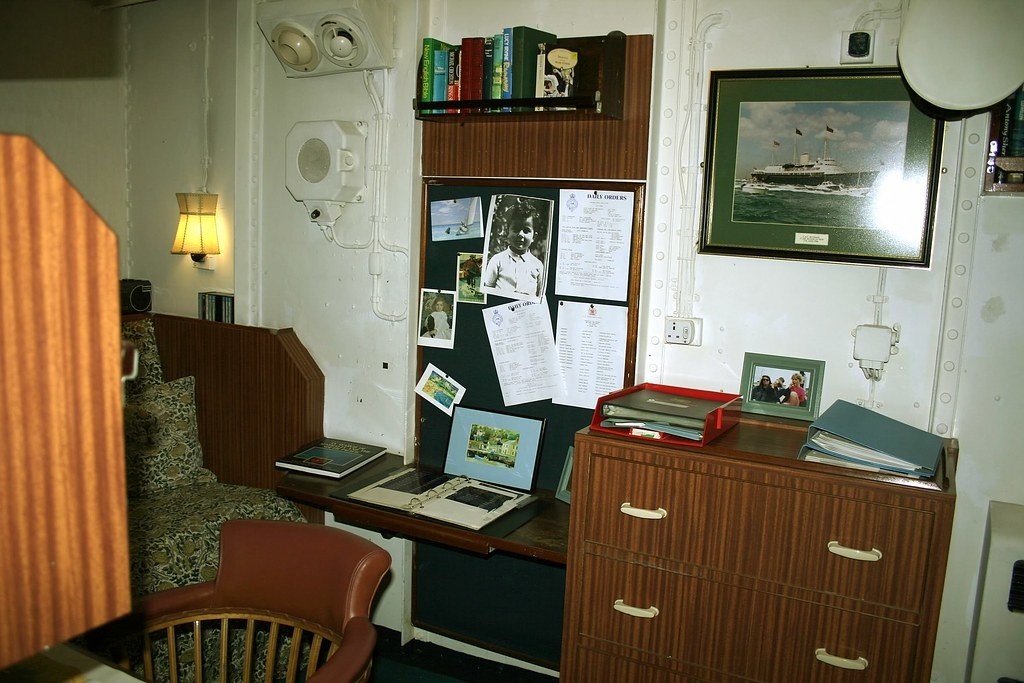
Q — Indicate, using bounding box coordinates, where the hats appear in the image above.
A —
[800,371,805,376]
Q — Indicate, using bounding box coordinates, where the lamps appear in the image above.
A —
[171,192,223,273]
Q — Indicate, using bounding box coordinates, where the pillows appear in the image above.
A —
[119,319,220,495]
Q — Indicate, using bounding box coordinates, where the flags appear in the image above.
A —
[795,128,803,136]
[774,141,780,146]
[826,126,834,133]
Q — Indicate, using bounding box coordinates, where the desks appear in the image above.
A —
[276,453,570,683]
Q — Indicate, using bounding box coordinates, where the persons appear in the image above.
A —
[463,254,480,291]
[751,373,806,407]
[421,297,452,340]
[484,202,543,297]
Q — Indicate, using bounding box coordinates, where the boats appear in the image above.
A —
[741,177,770,195]
[803,180,845,193]
[749,134,882,195]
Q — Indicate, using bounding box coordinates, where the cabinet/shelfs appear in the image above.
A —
[558,421,958,682]
[411,30,628,122]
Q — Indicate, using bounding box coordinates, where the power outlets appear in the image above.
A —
[665,315,704,347]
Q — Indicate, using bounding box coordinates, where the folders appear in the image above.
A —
[602,389,727,430]
[797,400,943,481]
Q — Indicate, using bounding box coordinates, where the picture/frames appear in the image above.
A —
[695,68,944,269]
[740,353,827,422]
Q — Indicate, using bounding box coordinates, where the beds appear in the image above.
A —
[68,310,324,683]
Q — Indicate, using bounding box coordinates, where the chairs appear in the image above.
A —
[132,520,393,683]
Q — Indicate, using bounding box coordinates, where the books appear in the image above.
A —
[273,436,388,482]
[996,90,1024,184]
[420,25,599,115]
[347,468,532,531]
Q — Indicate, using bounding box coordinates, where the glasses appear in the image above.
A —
[763,379,769,381]
